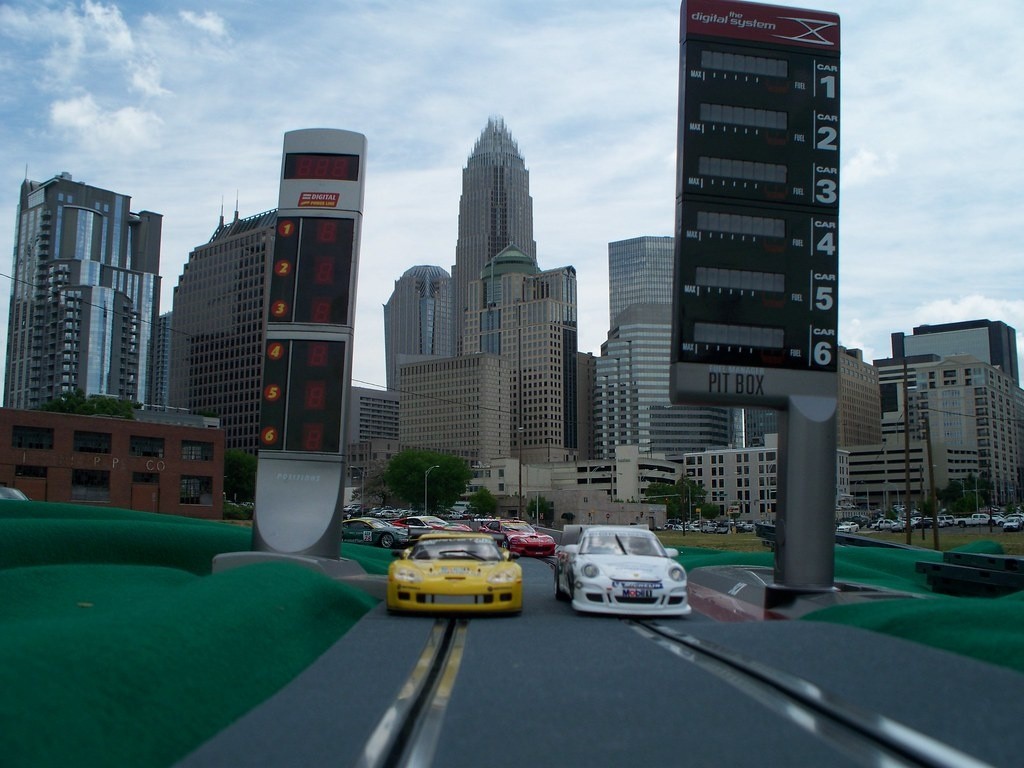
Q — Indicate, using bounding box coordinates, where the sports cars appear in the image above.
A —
[391,514,473,532]
[386,531,523,618]
[554,526,693,616]
[474,518,557,559]
[341,516,410,549]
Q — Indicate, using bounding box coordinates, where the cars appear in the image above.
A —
[835,505,1024,534]
[0,487,32,501]
[662,519,754,535]
[343,504,492,520]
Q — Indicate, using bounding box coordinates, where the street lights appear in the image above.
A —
[425,465,440,515]
[589,465,605,484]
[350,466,365,517]
[883,481,900,506]
[518,428,523,520]
[638,468,658,503]
